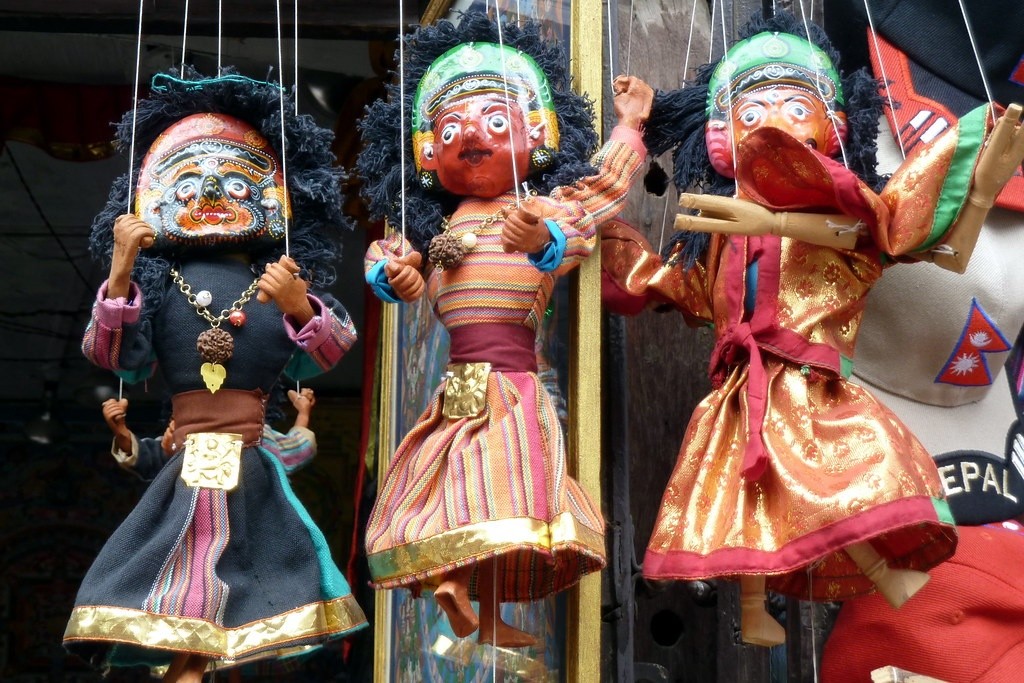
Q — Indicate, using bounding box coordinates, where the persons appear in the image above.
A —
[102,382,316,483]
[63,67,369,683]
[350,13,654,646]
[600,9,1024,645]
[429,197,527,270]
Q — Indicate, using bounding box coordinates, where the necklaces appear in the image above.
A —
[169,266,260,394]
